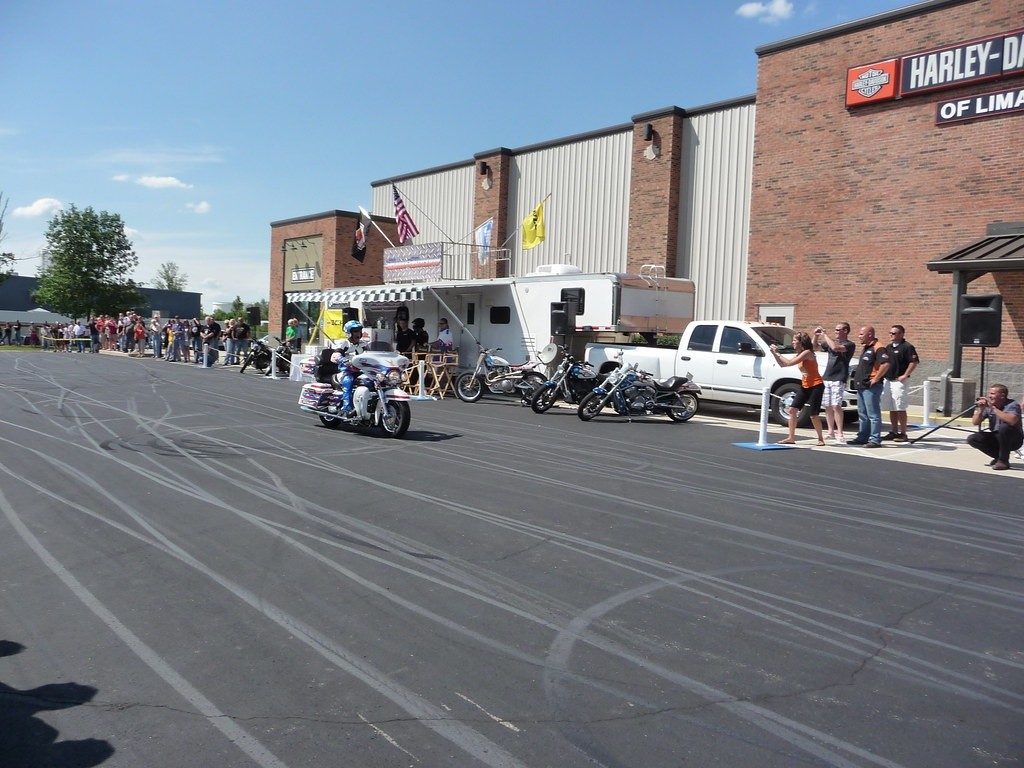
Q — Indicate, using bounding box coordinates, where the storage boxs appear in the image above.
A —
[298,382,332,410]
[299,358,316,374]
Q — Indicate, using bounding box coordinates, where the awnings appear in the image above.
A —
[285,282,515,304]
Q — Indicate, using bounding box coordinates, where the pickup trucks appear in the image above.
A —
[583,320,861,427]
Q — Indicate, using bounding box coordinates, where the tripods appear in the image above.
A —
[910,346,1024,460]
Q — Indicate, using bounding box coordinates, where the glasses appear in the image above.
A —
[889,331,900,336]
[351,329,362,333]
[439,323,446,325]
[835,328,844,332]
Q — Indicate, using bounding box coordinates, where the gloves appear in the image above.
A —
[342,359,352,367]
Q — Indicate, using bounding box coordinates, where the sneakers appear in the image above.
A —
[883,432,900,440]
[893,433,908,441]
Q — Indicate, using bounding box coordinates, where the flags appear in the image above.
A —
[351,208,372,263]
[522,201,545,250]
[474,216,493,266]
[393,183,420,246]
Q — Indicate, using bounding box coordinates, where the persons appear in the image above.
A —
[846,325,891,448]
[396,318,429,392]
[0,311,251,367]
[967,384,1023,470]
[436,318,453,352]
[285,318,304,354]
[811,322,856,445]
[330,320,370,412]
[880,326,920,442]
[769,322,825,448]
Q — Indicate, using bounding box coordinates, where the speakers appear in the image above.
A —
[246,307,261,326]
[342,307,358,331]
[958,294,1003,347]
[551,302,576,336]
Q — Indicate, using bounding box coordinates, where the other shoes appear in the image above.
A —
[993,459,1010,470]
[846,440,866,445]
[822,434,835,440]
[984,458,999,466]
[42,347,99,353]
[347,410,356,417]
[864,441,881,448]
[101,346,134,353]
[221,361,239,366]
[161,355,203,364]
[836,434,845,444]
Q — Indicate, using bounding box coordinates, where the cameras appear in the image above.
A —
[816,327,822,333]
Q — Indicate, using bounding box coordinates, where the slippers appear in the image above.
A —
[817,440,825,446]
[777,439,796,444]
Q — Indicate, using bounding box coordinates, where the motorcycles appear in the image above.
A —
[530,345,619,414]
[298,341,412,437]
[239,342,271,374]
[264,336,301,377]
[454,342,552,404]
[577,351,702,424]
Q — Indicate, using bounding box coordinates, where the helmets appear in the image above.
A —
[344,320,363,338]
[411,318,425,329]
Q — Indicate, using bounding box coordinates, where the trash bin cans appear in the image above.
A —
[928,376,941,413]
[947,378,976,418]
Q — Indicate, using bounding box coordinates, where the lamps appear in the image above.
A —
[479,161,489,175]
[643,124,654,140]
[279,242,291,251]
[300,237,314,247]
[290,240,300,250]
